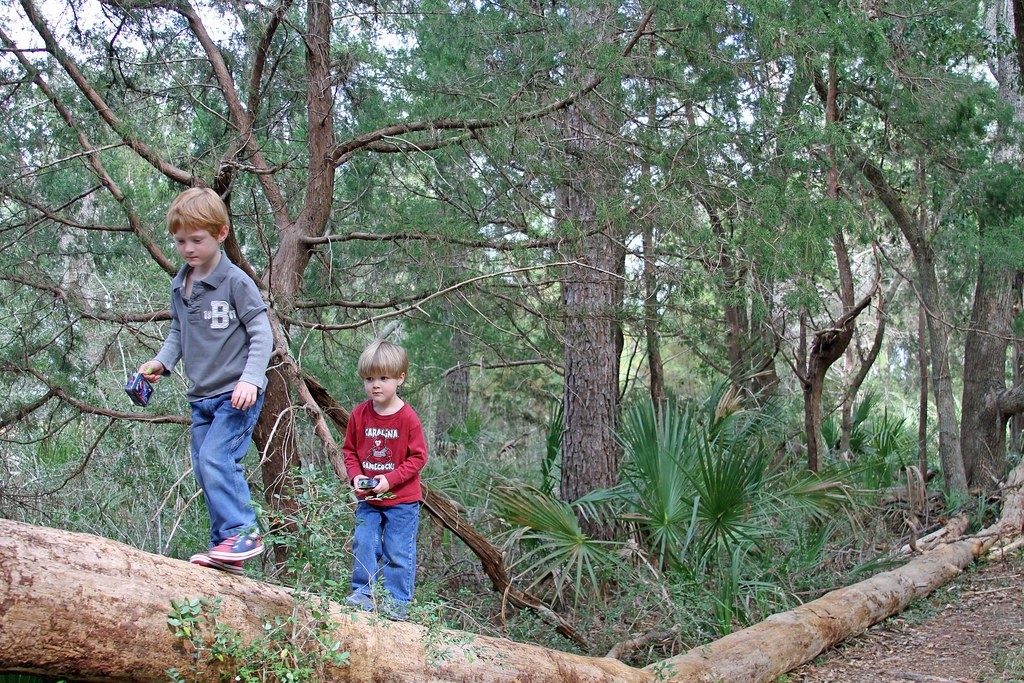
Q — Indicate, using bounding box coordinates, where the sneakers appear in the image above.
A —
[346,593,373,612]
[381,603,407,621]
[209,534,265,562]
[190,552,244,575]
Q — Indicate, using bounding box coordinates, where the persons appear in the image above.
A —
[343,341,427,621]
[138,187,274,575]
